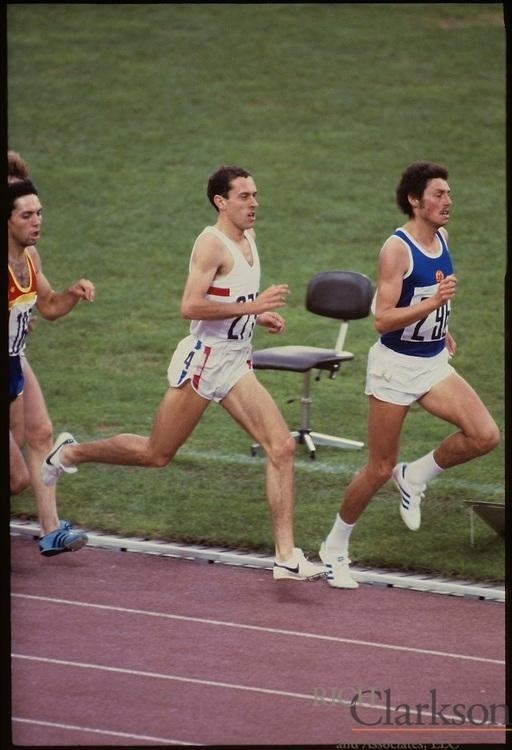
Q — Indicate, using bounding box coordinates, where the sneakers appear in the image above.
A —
[41,432,77,487]
[273,541,359,589]
[38,529,88,557]
[392,462,426,532]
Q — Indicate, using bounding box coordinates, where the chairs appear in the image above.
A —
[250,270,373,459]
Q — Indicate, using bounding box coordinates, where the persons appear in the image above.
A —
[38,161,335,583]
[9,148,90,561]
[316,159,506,590]
[6,177,98,496]
